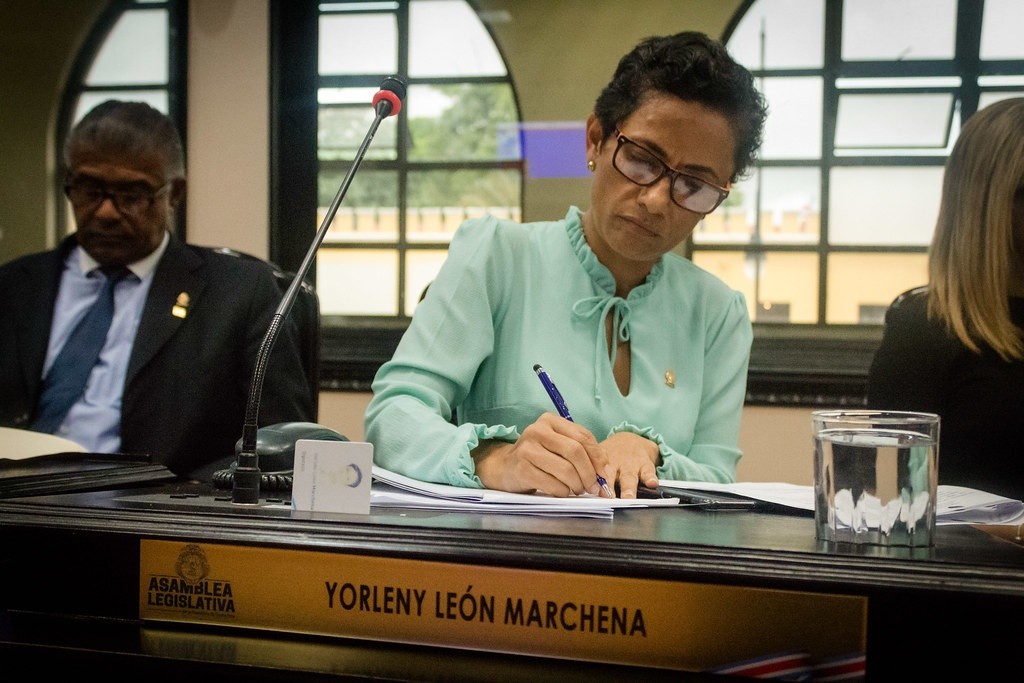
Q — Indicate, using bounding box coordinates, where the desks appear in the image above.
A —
[0,451,177,498]
[0,483,1024,683]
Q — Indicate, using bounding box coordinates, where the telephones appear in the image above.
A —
[212,420,354,493]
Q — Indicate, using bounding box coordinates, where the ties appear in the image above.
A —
[26,267,131,435]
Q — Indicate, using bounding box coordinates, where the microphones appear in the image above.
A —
[232,74,408,501]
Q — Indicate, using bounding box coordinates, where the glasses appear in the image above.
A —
[610,125,731,216]
[61,169,170,216]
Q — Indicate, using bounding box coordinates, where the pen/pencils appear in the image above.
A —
[533,361,615,498]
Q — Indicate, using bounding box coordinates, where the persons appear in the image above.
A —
[0,97,321,486]
[363,27,772,502]
[870,95,1022,500]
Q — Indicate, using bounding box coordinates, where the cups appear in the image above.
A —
[811,411,940,549]
